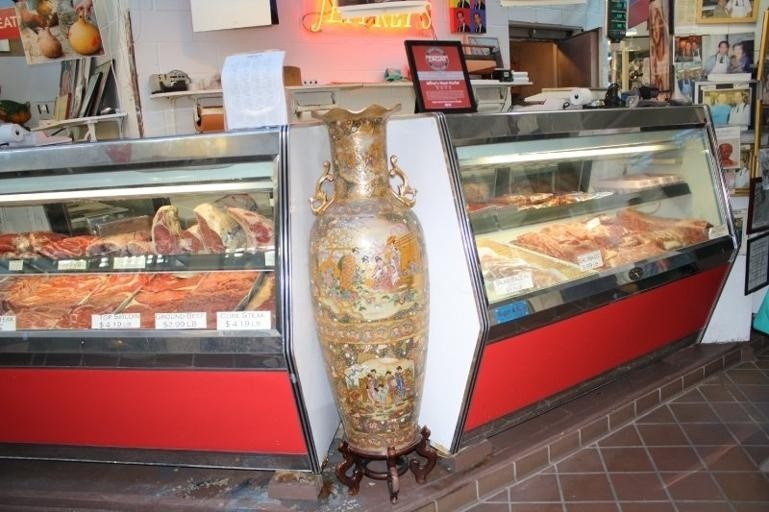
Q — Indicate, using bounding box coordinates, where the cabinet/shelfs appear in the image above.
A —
[386,105,742,454]
[0,122,339,476]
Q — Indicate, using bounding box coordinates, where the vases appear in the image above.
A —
[310,102,433,456]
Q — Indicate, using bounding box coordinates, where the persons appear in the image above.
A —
[458,0,470,8]
[727,91,750,124]
[74,0,93,20]
[724,0,752,17]
[729,42,753,74]
[474,0,485,10]
[333,238,405,402]
[711,93,731,124]
[472,12,486,33]
[735,158,749,188]
[705,41,730,73]
[719,144,737,166]
[455,11,470,32]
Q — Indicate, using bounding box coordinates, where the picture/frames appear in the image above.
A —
[53,58,118,119]
[742,231,768,295]
[746,176,769,235]
[404,38,479,114]
[648,1,675,93]
[696,0,760,23]
[697,81,757,132]
